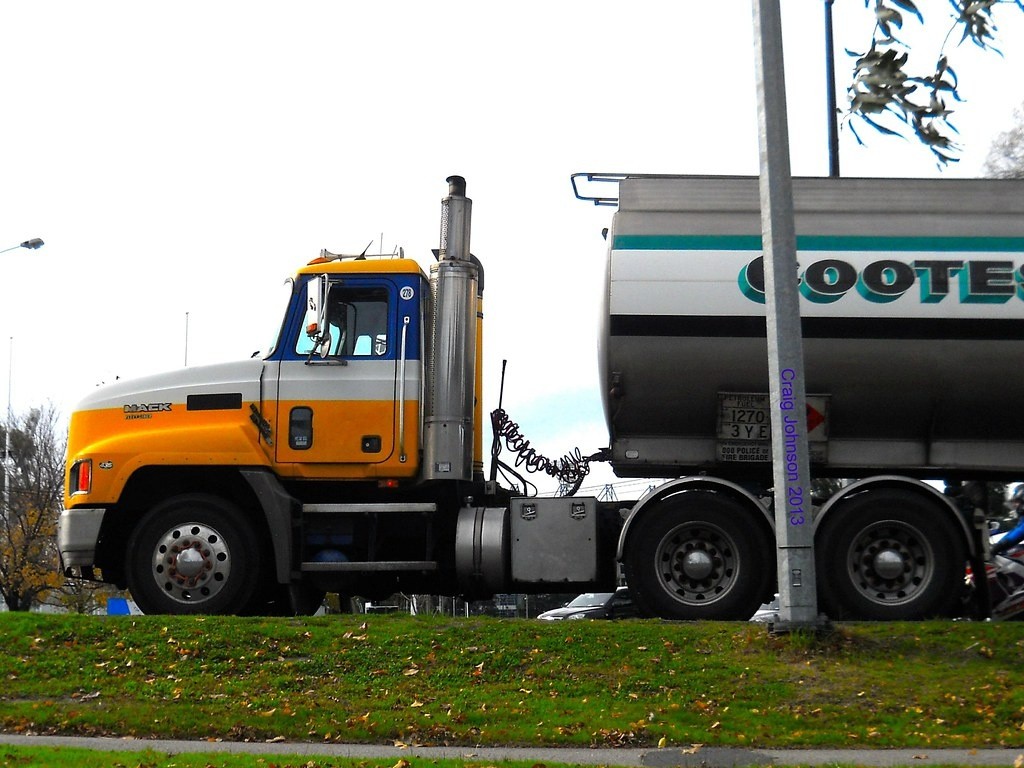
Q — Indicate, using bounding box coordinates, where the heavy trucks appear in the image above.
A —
[54,170,1024,626]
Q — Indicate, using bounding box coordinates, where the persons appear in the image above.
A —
[989,483,1024,558]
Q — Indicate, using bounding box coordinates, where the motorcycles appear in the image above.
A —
[964,516,1024,620]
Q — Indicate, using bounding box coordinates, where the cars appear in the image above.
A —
[749,592,780,621]
[536,586,635,622]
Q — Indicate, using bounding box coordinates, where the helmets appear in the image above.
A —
[1009,483,1024,514]
